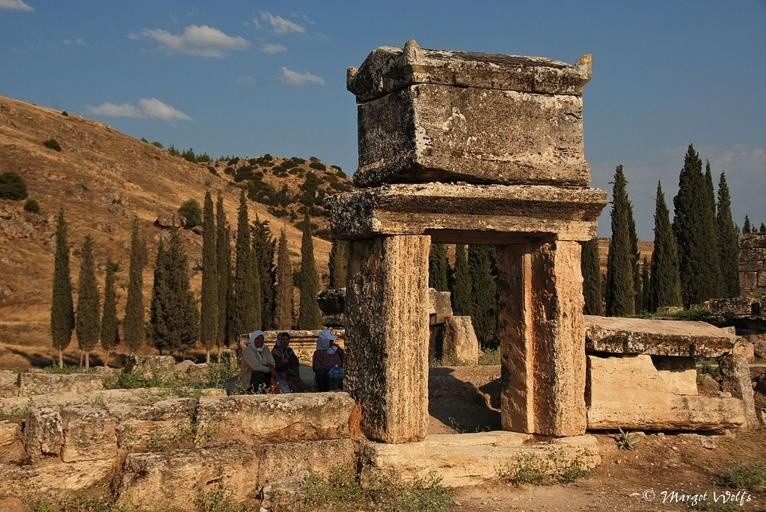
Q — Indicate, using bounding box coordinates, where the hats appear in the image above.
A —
[319,331,338,340]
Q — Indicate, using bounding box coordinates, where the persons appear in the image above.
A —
[271,332,312,393]
[313,329,346,393]
[241,330,275,394]
